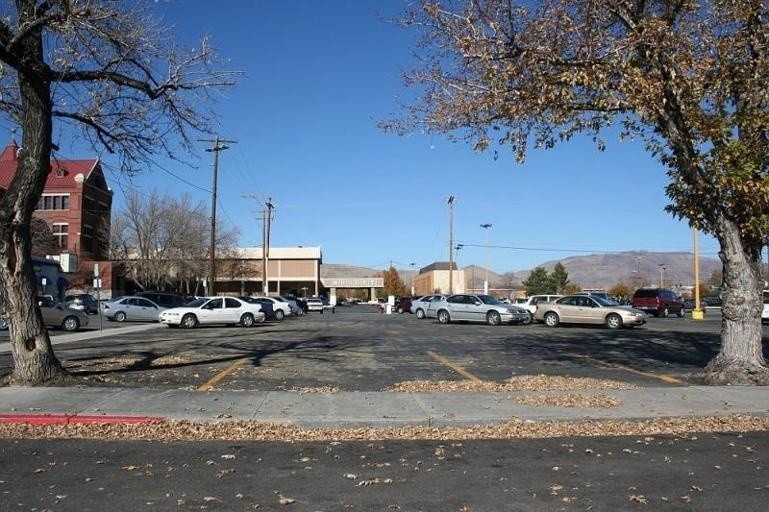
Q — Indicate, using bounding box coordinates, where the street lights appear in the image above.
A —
[480,222,494,297]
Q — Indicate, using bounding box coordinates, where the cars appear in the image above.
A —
[760,288,769,322]
